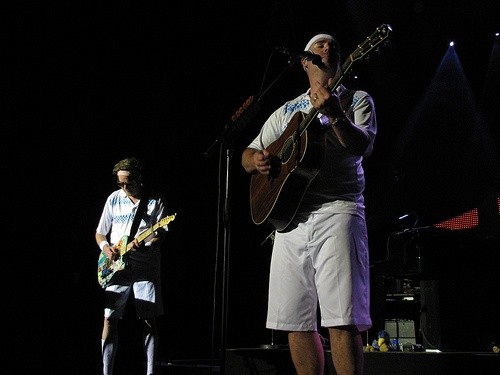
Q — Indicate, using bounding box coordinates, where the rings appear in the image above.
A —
[313,97,317,101]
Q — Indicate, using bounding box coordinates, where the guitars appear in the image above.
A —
[250,24,392,231]
[98,212,177,287]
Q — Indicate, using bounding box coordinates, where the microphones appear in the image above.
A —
[392,226,432,236]
[275,47,322,64]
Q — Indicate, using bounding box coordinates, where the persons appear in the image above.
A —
[94,158,170,375]
[241,34,378,375]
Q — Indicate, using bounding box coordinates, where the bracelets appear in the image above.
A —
[330,110,347,127]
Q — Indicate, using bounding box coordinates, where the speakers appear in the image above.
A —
[417,232,500,351]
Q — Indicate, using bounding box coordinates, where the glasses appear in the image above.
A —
[117,177,138,187]
